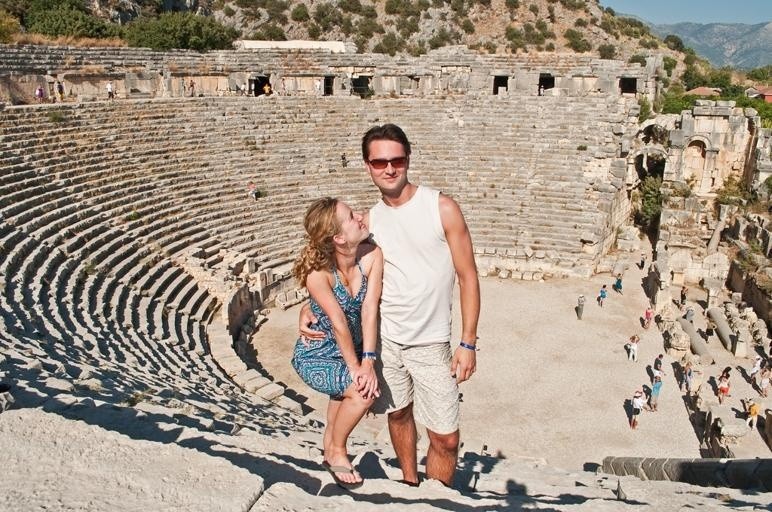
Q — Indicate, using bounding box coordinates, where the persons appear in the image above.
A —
[56,82,64,100]
[35,85,44,104]
[240,82,247,96]
[247,181,256,200]
[539,84,545,96]
[105,81,114,99]
[576,295,584,320]
[291,196,384,489]
[297,123,481,487]
[598,251,772,432]
[189,80,195,89]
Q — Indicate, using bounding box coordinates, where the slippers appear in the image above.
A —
[322,460,365,491]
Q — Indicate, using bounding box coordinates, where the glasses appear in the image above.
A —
[370,152,409,171]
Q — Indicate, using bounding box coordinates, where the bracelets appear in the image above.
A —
[362,351,377,361]
[459,340,477,350]
